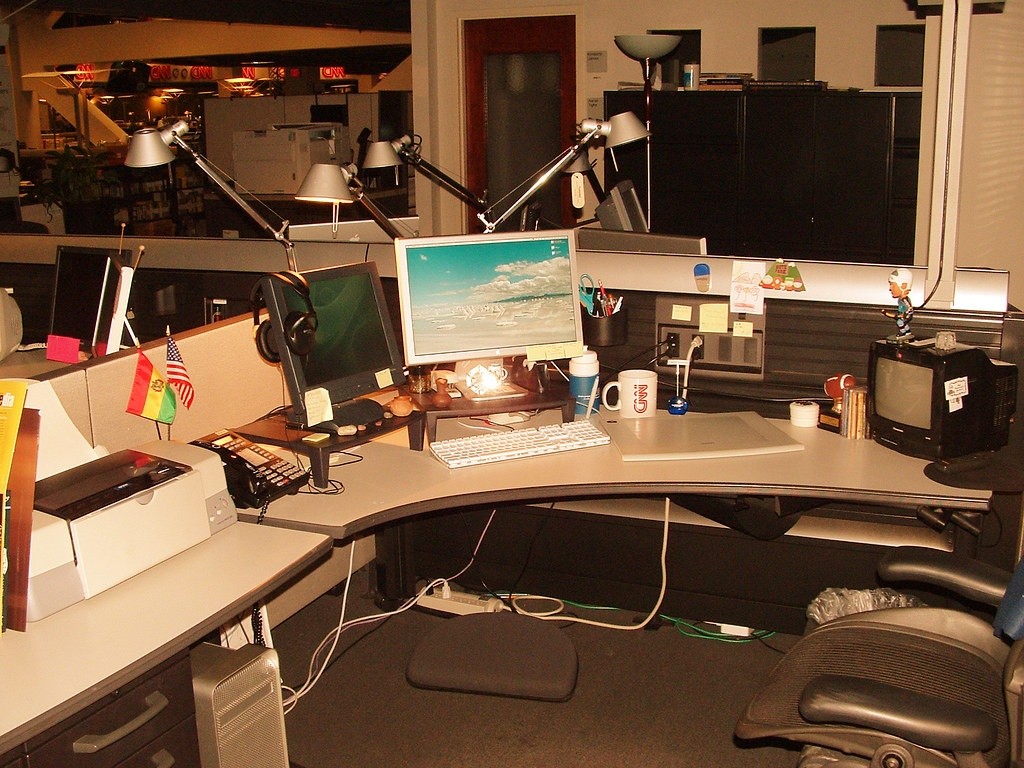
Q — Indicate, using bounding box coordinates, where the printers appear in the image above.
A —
[25,439,239,623]
[231,123,350,194]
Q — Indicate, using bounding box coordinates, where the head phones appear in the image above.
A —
[249,269,318,365]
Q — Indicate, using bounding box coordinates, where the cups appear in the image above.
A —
[601,370,659,418]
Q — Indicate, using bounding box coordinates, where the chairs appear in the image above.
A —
[733,526,1024,768]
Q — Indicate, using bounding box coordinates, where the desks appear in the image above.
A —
[234,404,992,613]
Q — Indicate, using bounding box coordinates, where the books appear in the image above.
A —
[840,385,871,441]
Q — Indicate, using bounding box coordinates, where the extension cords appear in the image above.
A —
[416,588,504,616]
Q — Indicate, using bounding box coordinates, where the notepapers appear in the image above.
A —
[302,432,330,443]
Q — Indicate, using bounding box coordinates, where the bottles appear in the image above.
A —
[568,350,600,415]
[683,64,700,91]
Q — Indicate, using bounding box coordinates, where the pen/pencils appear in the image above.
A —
[286,422,336,434]
[595,293,623,318]
[598,279,608,301]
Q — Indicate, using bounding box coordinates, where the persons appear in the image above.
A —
[882,268,914,338]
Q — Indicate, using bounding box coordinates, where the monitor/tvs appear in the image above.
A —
[577,180,707,255]
[263,260,407,433]
[47,245,135,359]
[866,340,1018,462]
[394,230,585,401]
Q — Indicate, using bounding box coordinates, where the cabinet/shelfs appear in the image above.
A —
[0,416,336,768]
[602,90,922,266]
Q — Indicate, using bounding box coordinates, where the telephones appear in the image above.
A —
[187,428,310,510]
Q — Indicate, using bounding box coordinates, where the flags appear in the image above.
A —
[167,337,193,410]
[126,346,177,425]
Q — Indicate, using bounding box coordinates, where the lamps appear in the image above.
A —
[475,111,652,235]
[294,161,424,239]
[361,131,497,230]
[612,35,683,231]
[124,120,301,272]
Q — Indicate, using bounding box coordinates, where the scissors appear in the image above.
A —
[579,274,594,314]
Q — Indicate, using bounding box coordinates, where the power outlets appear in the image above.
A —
[656,323,763,375]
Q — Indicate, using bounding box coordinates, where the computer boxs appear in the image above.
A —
[188,640,290,768]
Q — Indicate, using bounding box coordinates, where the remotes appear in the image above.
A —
[908,338,936,348]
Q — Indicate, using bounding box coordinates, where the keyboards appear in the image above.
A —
[430,420,611,469]
